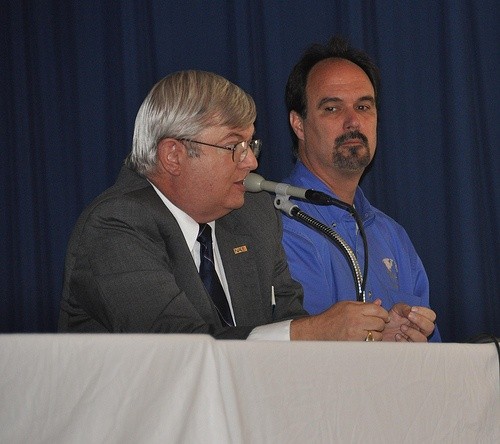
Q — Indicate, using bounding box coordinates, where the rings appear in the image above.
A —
[365,331,374,342]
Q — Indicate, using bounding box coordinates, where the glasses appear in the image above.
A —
[179,138,262,163]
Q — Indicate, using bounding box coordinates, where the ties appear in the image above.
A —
[196,223,236,328]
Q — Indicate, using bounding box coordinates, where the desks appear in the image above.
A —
[1,332,500,444]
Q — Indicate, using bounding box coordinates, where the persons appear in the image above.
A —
[278,53,440,342]
[54,70,436,343]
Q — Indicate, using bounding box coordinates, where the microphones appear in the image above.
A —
[243,173,332,204]
